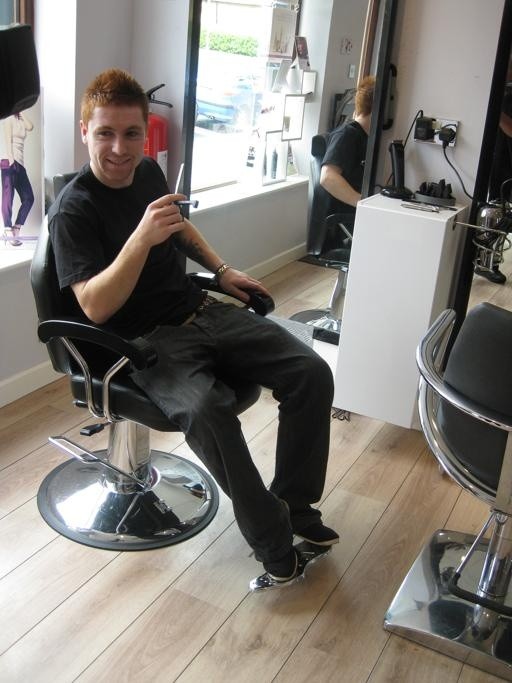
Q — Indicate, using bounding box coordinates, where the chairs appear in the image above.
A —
[290,131,357,345]
[384,302,512,683]
[28,213,332,593]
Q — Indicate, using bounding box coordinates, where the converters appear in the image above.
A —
[439,128,456,147]
[414,116,435,140]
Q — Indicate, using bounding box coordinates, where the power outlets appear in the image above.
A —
[435,119,459,147]
[413,115,438,145]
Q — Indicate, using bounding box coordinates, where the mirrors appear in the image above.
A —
[179,0,397,404]
[434,0,512,374]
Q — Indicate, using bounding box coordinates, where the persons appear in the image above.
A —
[472,72,512,284]
[319,75,377,246]
[45,66,342,584]
[295,36,308,58]
[0,107,36,248]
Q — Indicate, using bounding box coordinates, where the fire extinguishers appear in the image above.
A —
[144,83,174,184]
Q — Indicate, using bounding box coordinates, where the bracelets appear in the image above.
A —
[213,261,231,283]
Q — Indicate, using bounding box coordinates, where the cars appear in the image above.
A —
[194,47,252,133]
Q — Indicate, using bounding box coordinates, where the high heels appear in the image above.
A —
[3,229,22,246]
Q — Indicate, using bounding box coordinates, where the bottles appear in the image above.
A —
[268,148,278,179]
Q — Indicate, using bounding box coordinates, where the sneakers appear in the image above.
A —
[262,547,299,582]
[298,524,339,546]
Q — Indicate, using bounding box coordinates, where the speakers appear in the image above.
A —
[0,22,39,120]
